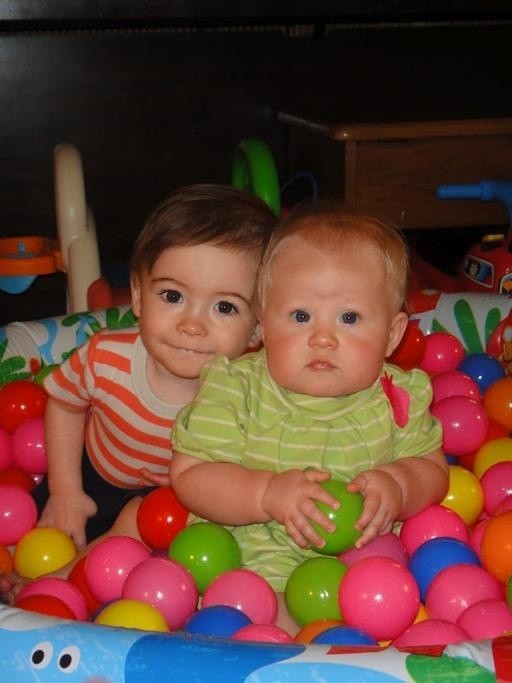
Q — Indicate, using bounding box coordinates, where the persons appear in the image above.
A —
[3,184,280,607]
[172,203,449,640]
